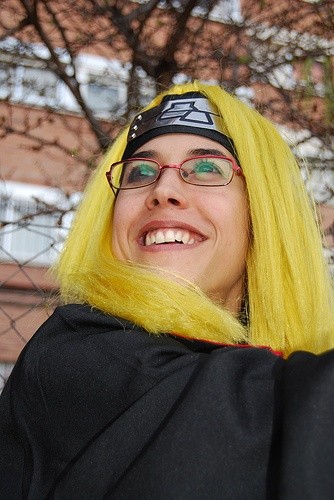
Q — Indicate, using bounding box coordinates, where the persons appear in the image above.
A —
[0,77,333,499]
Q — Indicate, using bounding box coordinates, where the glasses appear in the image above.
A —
[106,156,243,198]
